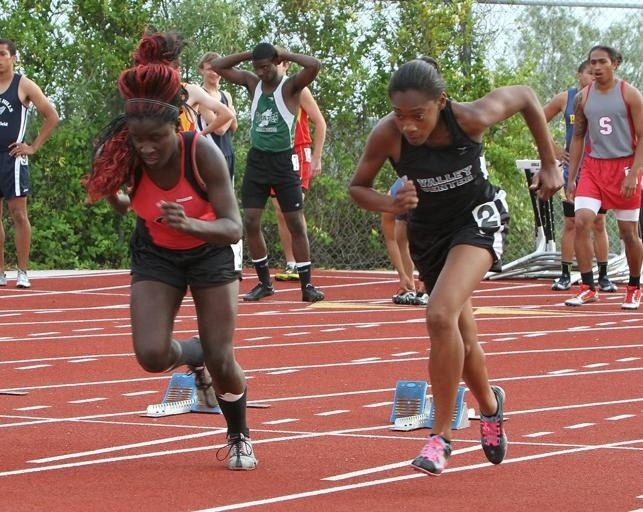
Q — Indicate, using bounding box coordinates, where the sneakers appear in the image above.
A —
[302,282,325,301]
[216,426,257,470]
[411,431,453,476]
[480,385,508,465]
[274,265,300,281]
[622,285,641,310]
[564,283,599,306]
[0,272,7,285]
[243,281,274,301]
[598,275,618,292]
[551,273,571,291]
[393,289,429,305]
[14,264,31,288]
[186,333,219,408]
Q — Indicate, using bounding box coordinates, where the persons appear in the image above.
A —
[540,61,619,295]
[567,46,642,309]
[80,35,258,472]
[378,172,430,305]
[347,58,567,476]
[208,43,326,303]
[0,40,59,288]
[192,50,238,183]
[268,59,326,281]
[172,58,236,140]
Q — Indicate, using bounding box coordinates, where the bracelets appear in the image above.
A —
[201,131,207,137]
